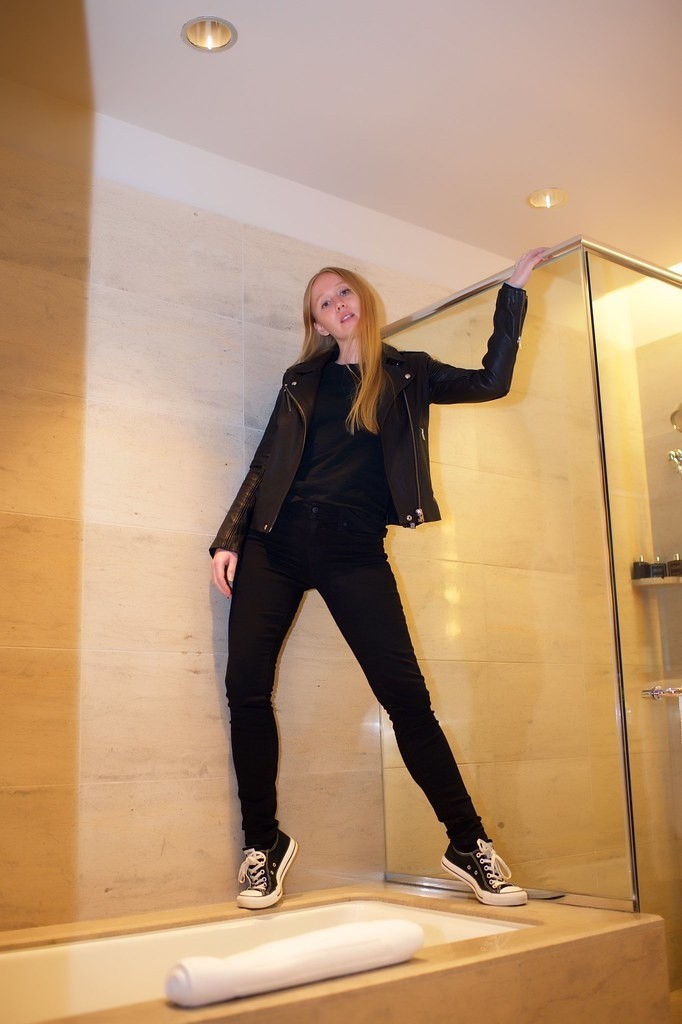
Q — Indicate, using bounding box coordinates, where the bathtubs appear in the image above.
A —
[0,877,670,1023]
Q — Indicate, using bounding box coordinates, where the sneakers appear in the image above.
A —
[440,840,530,907]
[236,828,299,911]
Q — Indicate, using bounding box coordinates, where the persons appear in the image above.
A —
[209,246,554,909]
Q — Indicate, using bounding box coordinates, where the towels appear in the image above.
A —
[165,918,424,1007]
